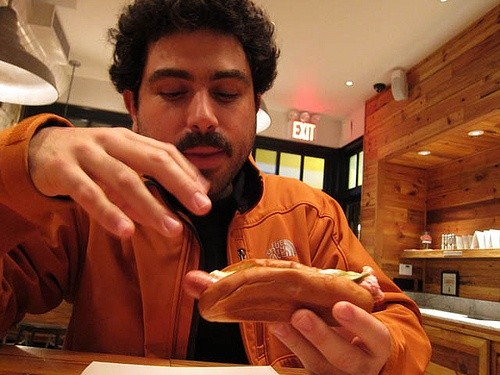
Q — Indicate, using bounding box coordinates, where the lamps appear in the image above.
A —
[256,98,272,135]
[299,111,311,123]
[288,109,299,121]
[311,114,321,124]
[0,0,59,107]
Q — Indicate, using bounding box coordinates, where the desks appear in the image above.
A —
[0,343,311,374]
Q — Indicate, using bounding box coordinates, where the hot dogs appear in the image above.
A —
[182,258,385,323]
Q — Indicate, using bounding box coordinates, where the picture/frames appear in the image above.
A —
[440,271,460,297]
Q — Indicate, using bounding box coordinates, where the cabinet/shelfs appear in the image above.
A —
[399,247,500,375]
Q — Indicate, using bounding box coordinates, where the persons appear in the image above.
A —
[0,0,433,375]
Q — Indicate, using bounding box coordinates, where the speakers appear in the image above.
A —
[391,70,408,101]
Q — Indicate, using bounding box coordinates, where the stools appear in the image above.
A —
[14,322,68,348]
